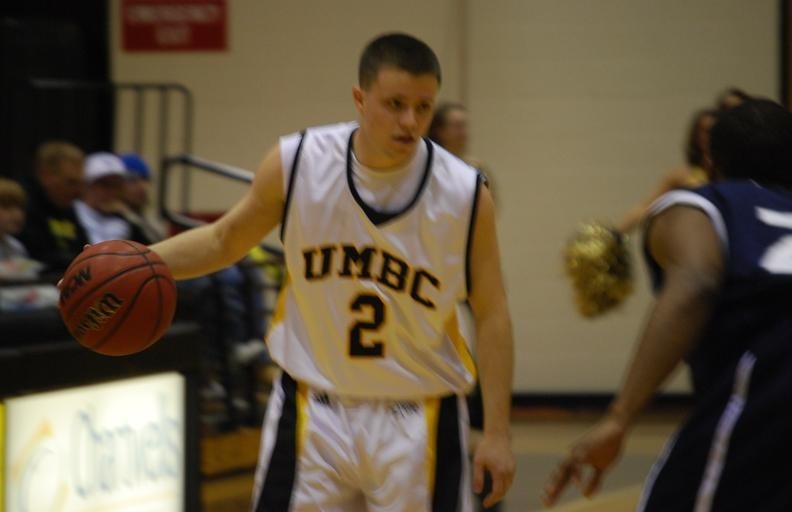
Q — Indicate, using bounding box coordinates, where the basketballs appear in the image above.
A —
[59,240,176,356]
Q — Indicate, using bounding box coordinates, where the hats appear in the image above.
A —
[81,152,149,187]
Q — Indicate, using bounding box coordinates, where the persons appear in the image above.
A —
[432,102,490,189]
[614,85,749,238]
[543,98,792,511]
[58,31,517,511]
